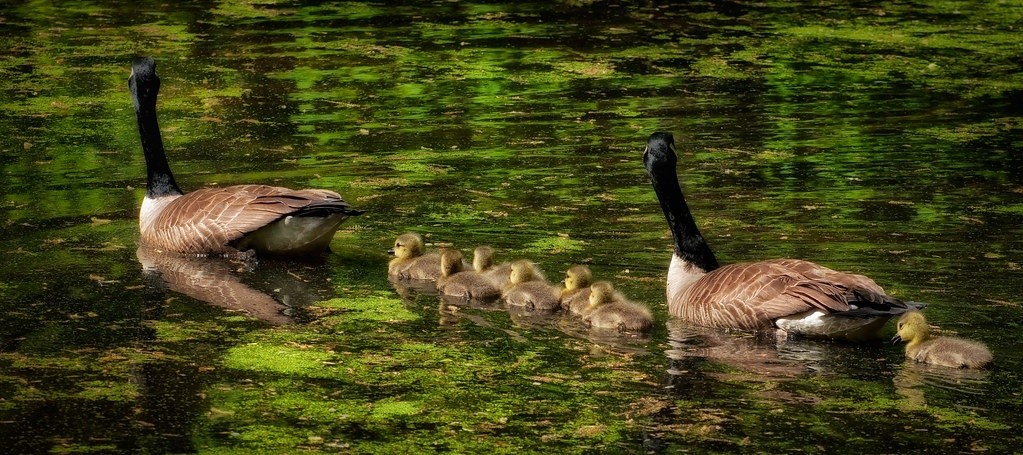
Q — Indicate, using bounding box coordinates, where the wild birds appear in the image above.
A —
[127,51,365,265]
[384,233,654,334]
[642,130,927,345]
[891,310,995,370]
